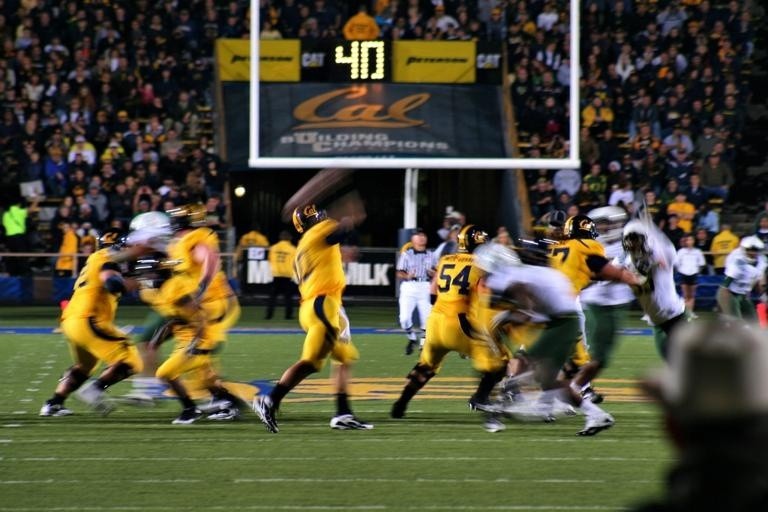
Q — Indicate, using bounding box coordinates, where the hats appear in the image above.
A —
[410,228,425,236]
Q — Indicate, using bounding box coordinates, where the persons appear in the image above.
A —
[625,310,767,511]
[264,230,298,319]
[251,167,374,433]
[0,0,244,268]
[231,230,270,278]
[42,184,243,427]
[228,1,484,42]
[388,0,767,316]
[389,207,684,437]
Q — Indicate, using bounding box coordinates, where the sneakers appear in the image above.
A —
[40,399,73,417]
[205,406,240,422]
[469,395,503,414]
[391,401,407,418]
[250,395,280,433]
[330,414,374,430]
[405,337,419,354]
[172,408,203,424]
[575,413,615,437]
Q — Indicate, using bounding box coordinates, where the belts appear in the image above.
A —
[413,277,428,281]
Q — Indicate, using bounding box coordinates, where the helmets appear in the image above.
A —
[292,203,328,233]
[590,206,627,242]
[563,216,598,239]
[99,231,123,245]
[740,235,764,264]
[136,251,171,288]
[166,200,208,229]
[457,224,489,252]
